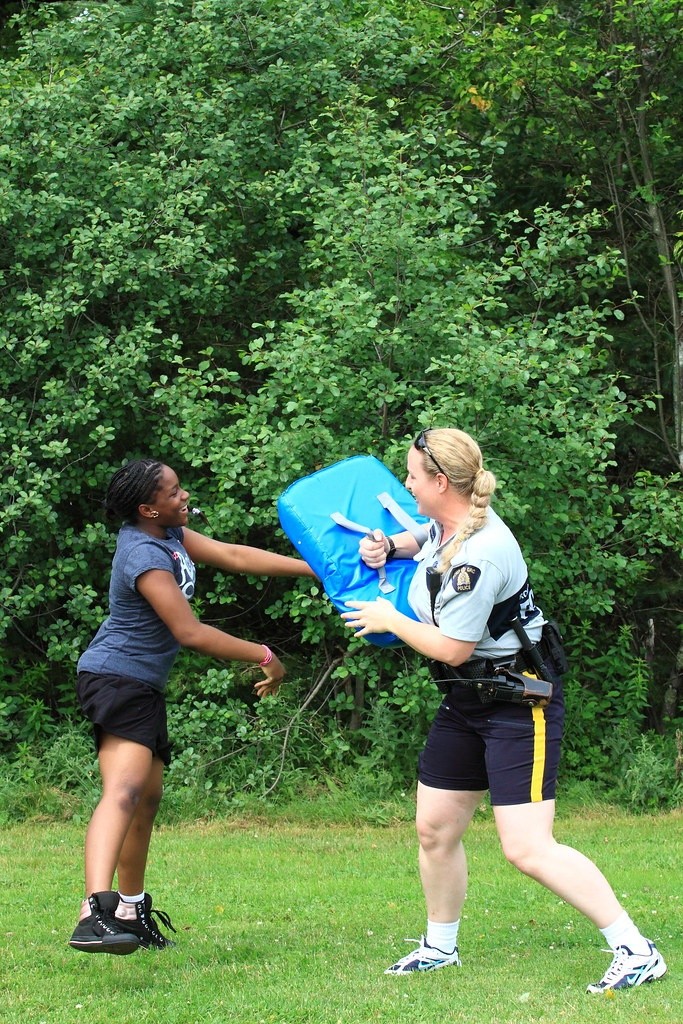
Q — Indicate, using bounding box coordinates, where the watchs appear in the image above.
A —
[385,532,396,563]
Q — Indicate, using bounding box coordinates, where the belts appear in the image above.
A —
[513,641,549,674]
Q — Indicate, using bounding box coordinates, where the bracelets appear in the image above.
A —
[258,641,273,667]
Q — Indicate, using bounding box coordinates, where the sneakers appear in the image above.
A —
[585,939,669,997]
[67,891,140,957]
[384,934,462,979]
[113,889,178,953]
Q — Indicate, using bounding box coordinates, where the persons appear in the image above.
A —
[345,427,666,994]
[68,457,319,953]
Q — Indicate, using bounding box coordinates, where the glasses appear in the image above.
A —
[414,428,451,482]
